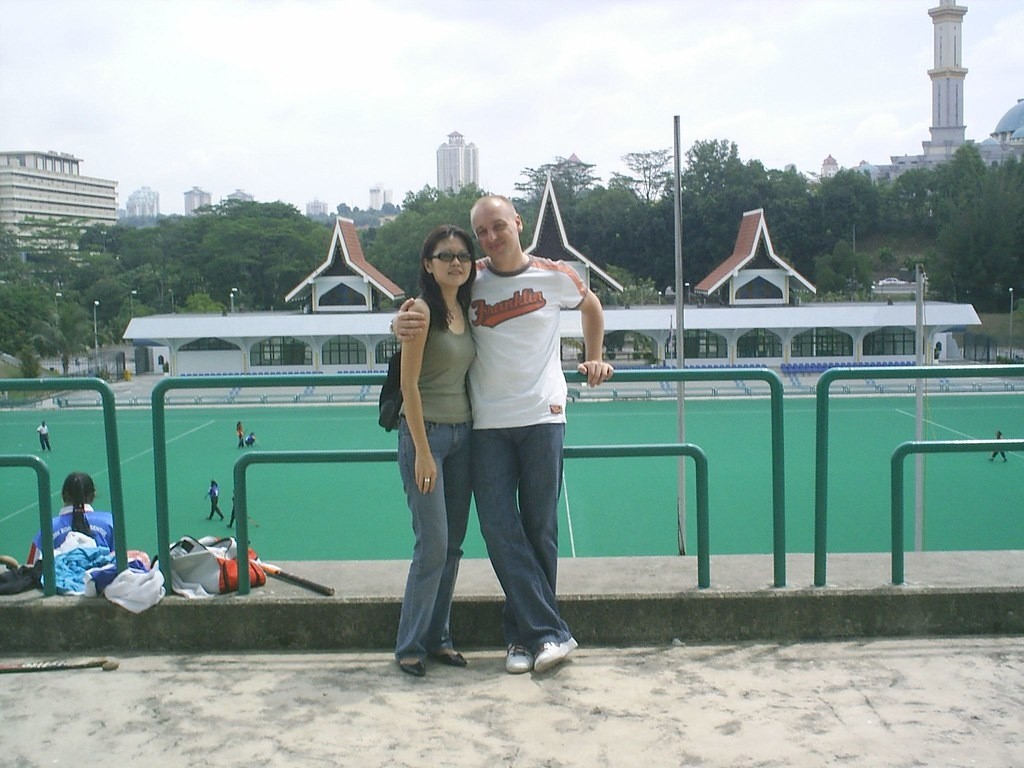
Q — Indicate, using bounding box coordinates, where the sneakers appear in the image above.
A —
[506,640,533,673]
[534,635,578,674]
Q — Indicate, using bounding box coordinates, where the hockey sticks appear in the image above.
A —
[259,562,337,597]
[1,655,120,674]
[247,515,258,527]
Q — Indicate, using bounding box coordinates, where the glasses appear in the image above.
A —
[427,251,472,263]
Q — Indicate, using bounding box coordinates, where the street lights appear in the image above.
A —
[1009,287,1014,358]
[54,292,63,327]
[685,282,690,306]
[93,300,100,373]
[130,290,137,318]
[229,287,238,313]
[168,289,174,312]
[658,291,662,305]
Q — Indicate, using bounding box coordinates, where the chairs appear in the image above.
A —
[180,371,388,376]
[781,361,916,377]
[614,364,767,369]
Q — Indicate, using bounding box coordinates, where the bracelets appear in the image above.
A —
[390,318,394,332]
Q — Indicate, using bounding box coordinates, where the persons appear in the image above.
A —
[394,223,477,677]
[989,430,1008,463]
[390,198,616,674]
[37,422,52,451]
[245,432,256,447]
[26,473,116,563]
[204,480,224,521]
[236,421,244,449]
[227,489,235,528]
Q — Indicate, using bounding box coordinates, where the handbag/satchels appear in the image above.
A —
[150,536,266,593]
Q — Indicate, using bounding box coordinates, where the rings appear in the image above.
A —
[424,478,430,482]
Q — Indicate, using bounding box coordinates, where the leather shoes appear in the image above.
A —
[428,651,467,667]
[400,657,426,676]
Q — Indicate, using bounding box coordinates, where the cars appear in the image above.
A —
[879,278,904,283]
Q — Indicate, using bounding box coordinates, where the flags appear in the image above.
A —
[668,330,672,347]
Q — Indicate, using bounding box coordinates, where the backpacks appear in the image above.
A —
[378,350,404,432]
